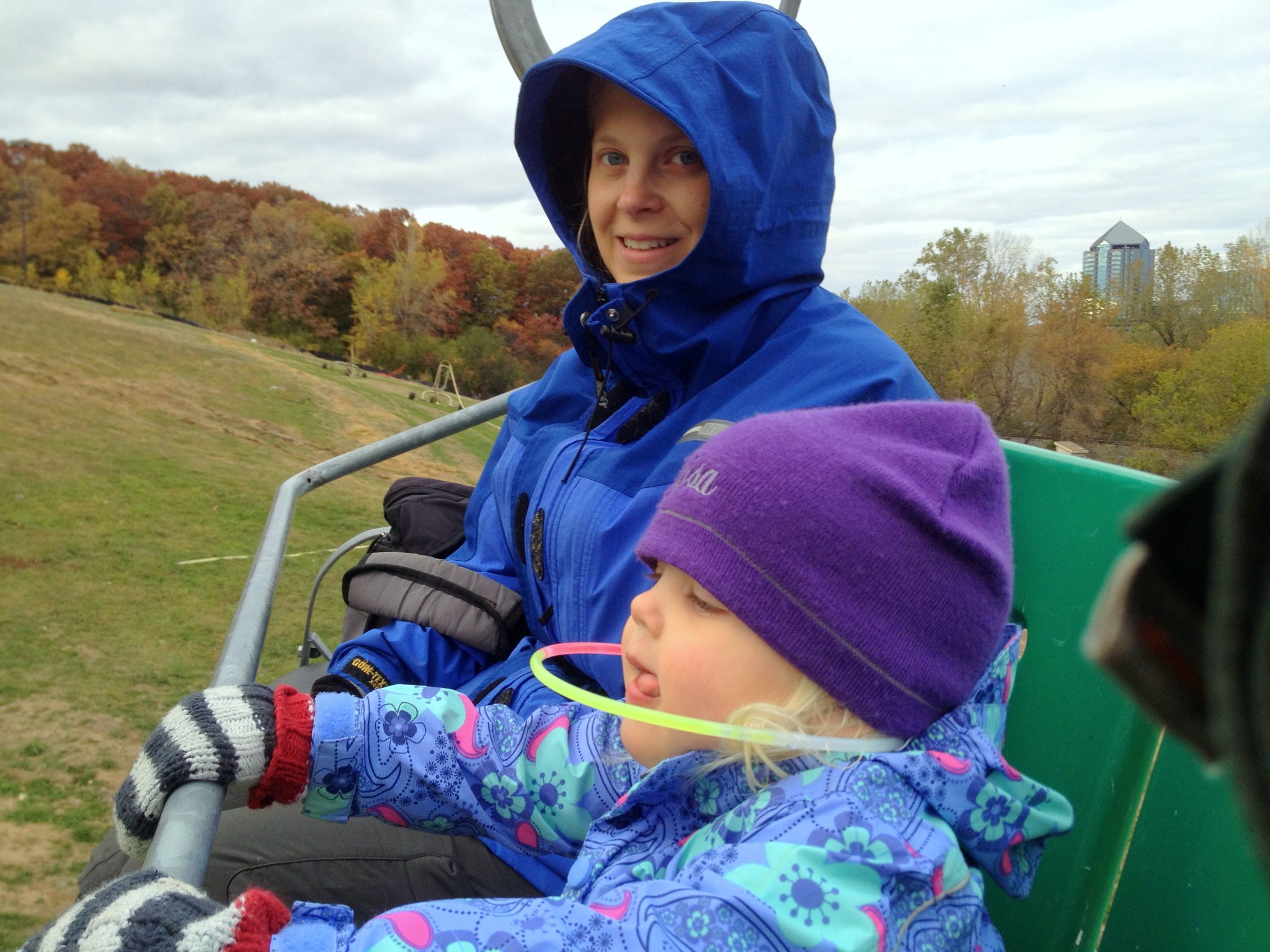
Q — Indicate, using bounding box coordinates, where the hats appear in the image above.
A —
[636,399,1014,735]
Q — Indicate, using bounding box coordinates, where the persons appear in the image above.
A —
[21,398,1073,952]
[80,0,938,921]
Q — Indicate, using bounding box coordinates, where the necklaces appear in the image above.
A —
[529,642,903,752]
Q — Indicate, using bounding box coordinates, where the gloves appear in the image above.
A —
[16,871,290,952]
[113,684,274,863]
